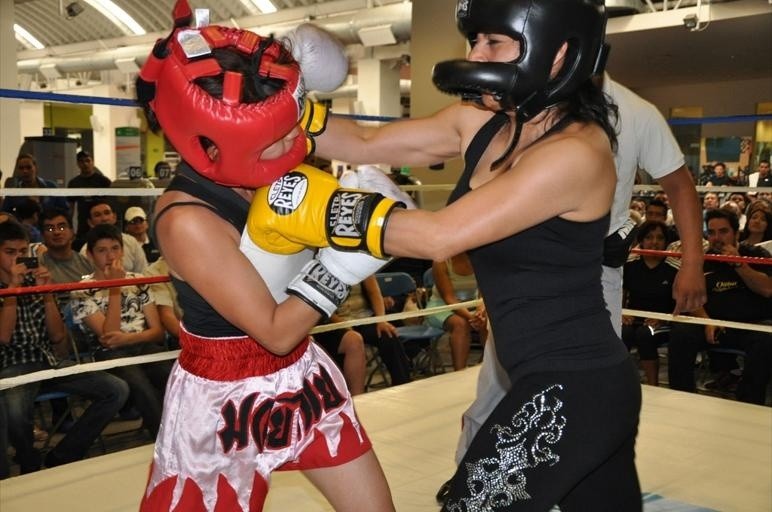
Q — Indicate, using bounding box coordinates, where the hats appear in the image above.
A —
[76,150,90,161]
[123,206,146,222]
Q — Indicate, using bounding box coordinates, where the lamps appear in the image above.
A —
[683,13,700,33]
[64,2,85,21]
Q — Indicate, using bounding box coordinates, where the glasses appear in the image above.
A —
[130,220,145,225]
[45,222,69,232]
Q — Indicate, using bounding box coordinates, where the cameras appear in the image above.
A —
[16,257,39,269]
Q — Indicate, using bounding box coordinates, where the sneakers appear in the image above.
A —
[705,375,734,389]
[44,451,67,468]
[121,405,140,420]
[20,463,41,474]
[53,422,76,431]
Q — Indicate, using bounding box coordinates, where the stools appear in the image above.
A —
[35,387,108,454]
[699,346,745,389]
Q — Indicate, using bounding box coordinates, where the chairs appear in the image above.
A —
[364,271,445,395]
[422,267,450,368]
[64,302,132,420]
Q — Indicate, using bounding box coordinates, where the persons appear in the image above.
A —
[312,164,488,395]
[132,2,419,511]
[239,2,648,510]
[0,150,185,478]
[451,65,710,482]
[617,154,771,408]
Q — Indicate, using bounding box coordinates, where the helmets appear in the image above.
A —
[139,0,307,187]
[432,0,611,118]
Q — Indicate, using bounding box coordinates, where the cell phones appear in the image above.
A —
[715,331,722,342]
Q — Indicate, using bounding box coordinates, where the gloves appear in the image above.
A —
[300,93,329,155]
[284,23,347,93]
[285,164,417,322]
[247,162,409,260]
